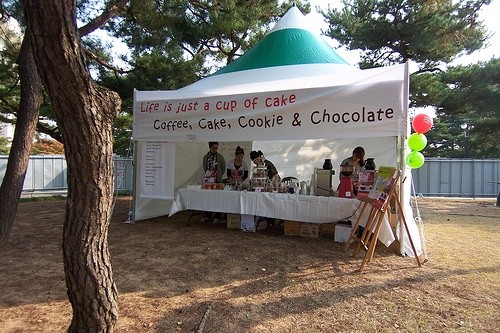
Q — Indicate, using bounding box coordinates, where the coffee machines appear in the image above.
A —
[338,165,356,198]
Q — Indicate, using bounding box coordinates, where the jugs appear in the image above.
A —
[364,158,376,170]
[323,159,332,170]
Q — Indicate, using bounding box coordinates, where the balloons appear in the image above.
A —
[406,114,433,169]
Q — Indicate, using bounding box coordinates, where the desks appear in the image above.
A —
[168,187,396,248]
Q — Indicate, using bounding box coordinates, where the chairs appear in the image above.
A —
[281,176,299,194]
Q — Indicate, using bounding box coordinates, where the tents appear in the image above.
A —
[132,7,410,256]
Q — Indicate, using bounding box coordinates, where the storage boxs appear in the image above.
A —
[241,214,266,233]
[227,214,241,229]
[283,220,300,236]
[300,224,324,238]
[322,224,335,234]
[335,225,358,244]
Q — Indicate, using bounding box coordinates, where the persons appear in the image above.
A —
[203,142,226,183]
[337,147,365,197]
[226,145,249,186]
[250,150,281,191]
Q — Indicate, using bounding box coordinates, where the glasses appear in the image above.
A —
[211,147,218,149]
